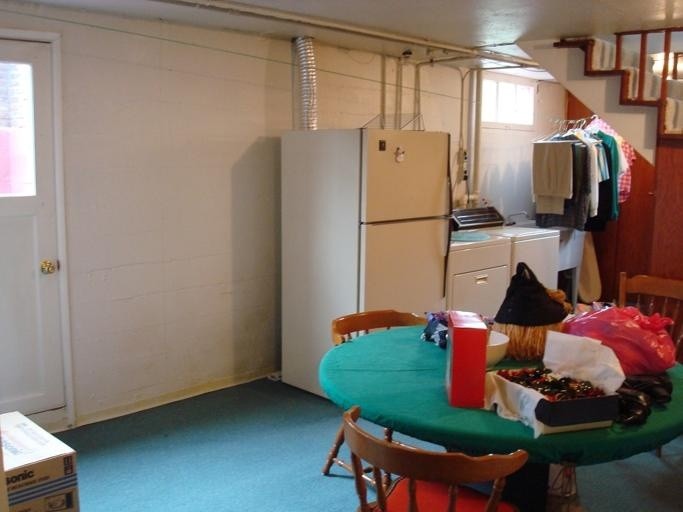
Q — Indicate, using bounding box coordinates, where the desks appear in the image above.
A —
[317,324,683,512]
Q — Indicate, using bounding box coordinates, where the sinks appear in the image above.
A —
[515,220,585,272]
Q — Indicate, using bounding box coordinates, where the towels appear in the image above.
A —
[533,142,574,217]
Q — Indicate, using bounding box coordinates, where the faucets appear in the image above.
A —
[505,212,530,223]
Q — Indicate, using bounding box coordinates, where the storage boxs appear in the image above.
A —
[0,410,80,512]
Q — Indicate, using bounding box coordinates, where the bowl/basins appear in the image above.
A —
[486,329,510,371]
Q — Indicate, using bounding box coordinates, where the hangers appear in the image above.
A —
[532,114,606,146]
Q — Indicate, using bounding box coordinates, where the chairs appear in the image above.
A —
[340,403,528,509]
[323,308,444,491]
[617,270,683,458]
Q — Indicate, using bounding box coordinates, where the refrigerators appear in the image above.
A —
[282,129,449,401]
